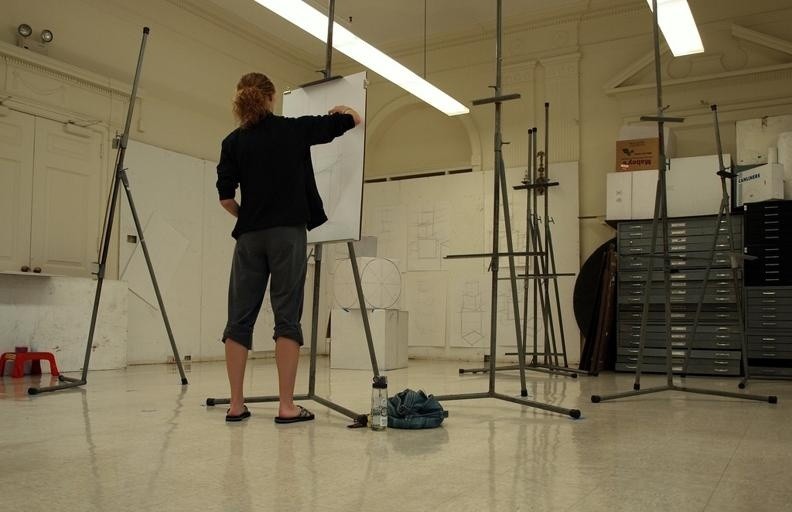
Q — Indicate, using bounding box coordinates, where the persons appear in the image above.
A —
[216,73,360,424]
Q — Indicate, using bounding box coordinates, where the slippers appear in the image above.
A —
[274,404,315,423]
[225,405,251,421]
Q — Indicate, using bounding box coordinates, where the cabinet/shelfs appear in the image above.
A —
[614,200,792,373]
[0,98,108,278]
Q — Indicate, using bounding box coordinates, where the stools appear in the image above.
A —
[0,351,59,379]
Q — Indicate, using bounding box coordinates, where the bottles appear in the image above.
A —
[370,383,388,430]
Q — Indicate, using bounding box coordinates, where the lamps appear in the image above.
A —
[16,21,53,45]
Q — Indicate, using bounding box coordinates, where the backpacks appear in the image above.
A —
[347,388,448,428]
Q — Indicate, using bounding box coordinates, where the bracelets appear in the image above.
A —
[343,108,353,115]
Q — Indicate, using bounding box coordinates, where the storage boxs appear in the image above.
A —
[323,308,409,370]
[615,137,662,170]
[735,163,784,209]
